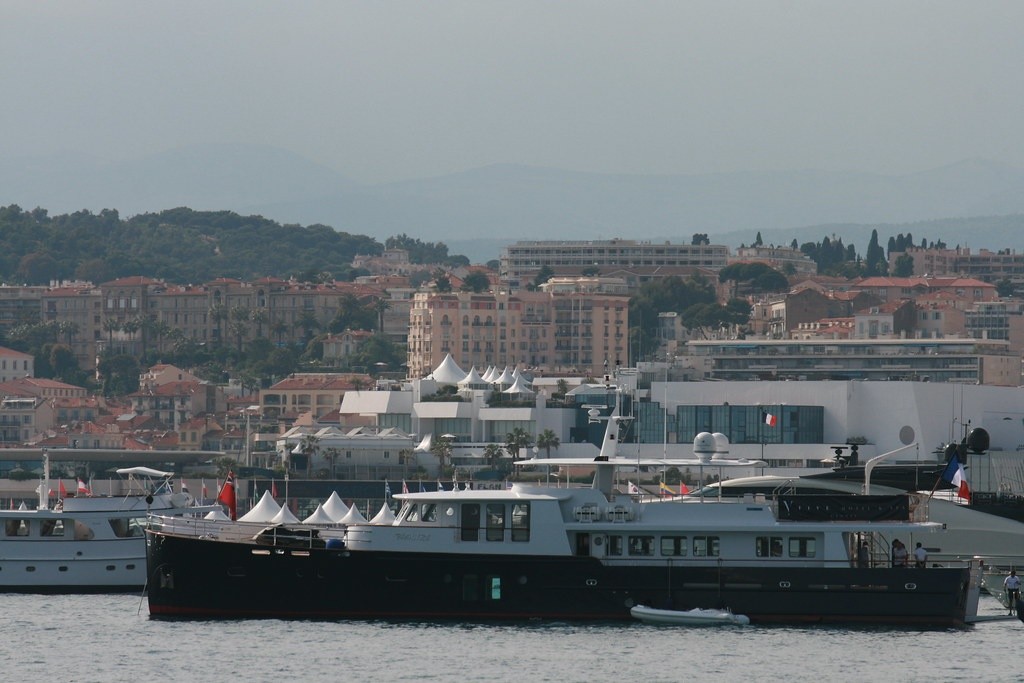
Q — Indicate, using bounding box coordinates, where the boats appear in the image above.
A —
[0,463,223,595]
[250,523,326,550]
[630,603,751,627]
[134,385,996,631]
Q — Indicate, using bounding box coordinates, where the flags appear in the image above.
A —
[629,482,644,497]
[219,469,236,521]
[660,482,675,496]
[79,479,90,493]
[386,481,443,494]
[762,412,776,426]
[60,480,66,496]
[203,480,222,497]
[942,450,970,499]
[182,480,189,493]
[681,482,688,494]
[49,489,54,496]
[237,477,276,498]
[11,499,14,508]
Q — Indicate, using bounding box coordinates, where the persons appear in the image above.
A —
[630,539,638,551]
[1004,571,1020,609]
[892,539,906,567]
[862,541,868,559]
[914,543,927,568]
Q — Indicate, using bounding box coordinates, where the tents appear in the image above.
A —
[423,352,535,407]
